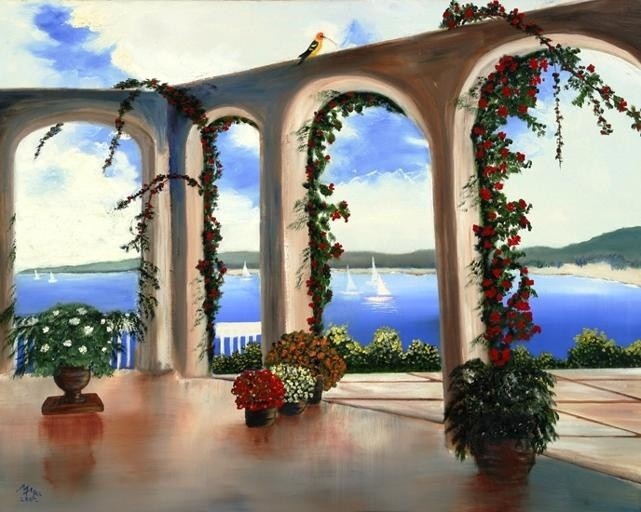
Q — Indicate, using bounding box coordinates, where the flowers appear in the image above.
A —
[223,329,347,410]
[6,301,134,380]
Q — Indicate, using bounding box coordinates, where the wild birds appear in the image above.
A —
[298,30,339,66]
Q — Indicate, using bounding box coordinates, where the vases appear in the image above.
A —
[244,401,299,427]
[55,364,91,403]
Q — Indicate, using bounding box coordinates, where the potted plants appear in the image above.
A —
[443,346,560,481]
